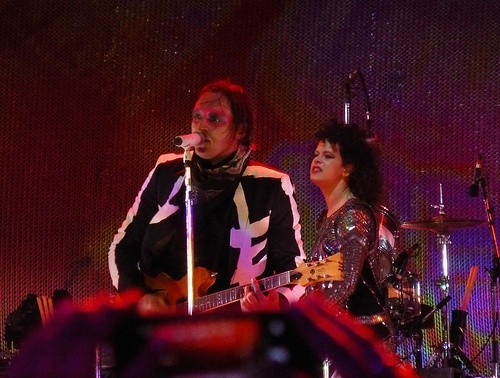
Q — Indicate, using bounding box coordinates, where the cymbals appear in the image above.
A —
[401,215,489,233]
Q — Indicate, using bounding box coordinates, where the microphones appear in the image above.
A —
[392,244,419,275]
[344,80,351,125]
[469,155,483,198]
[174,131,206,147]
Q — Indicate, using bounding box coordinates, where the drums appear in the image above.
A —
[387,278,422,320]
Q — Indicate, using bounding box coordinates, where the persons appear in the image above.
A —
[307,122,391,378]
[107,80,306,318]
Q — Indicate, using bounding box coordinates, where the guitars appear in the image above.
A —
[145,252,345,313]
